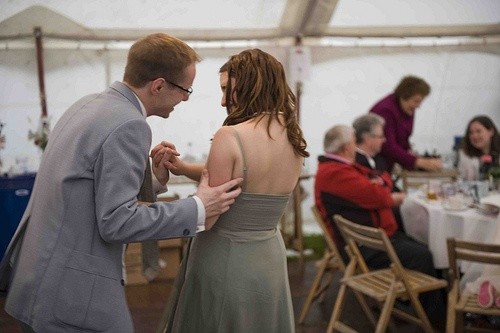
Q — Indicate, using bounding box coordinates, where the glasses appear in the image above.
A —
[370,134,385,139]
[152,76,193,95]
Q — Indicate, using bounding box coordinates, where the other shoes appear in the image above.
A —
[477,282,500,308]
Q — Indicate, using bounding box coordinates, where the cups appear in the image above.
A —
[427,177,473,210]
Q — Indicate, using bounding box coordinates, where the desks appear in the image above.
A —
[399,178,500,273]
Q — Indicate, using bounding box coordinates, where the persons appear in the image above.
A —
[3,33,243,333]
[315,76,444,321]
[464,114,500,182]
[150,47,309,333]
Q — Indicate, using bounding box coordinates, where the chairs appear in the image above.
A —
[326,213,448,333]
[296,204,397,331]
[276,179,304,297]
[445,236,500,333]
